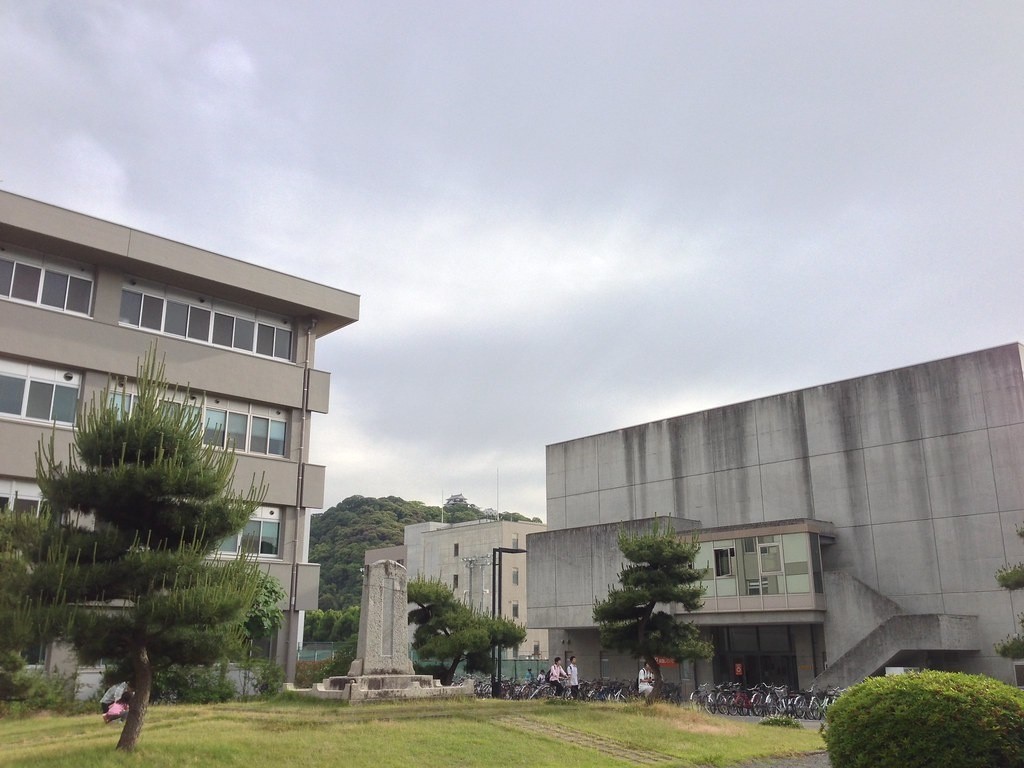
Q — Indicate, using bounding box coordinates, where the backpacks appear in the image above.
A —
[545,669,551,683]
[636,676,638,691]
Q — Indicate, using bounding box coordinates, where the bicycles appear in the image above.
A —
[453,674,840,720]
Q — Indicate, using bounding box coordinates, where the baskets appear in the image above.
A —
[560,678,572,689]
[775,688,788,698]
[805,692,812,700]
[817,690,825,700]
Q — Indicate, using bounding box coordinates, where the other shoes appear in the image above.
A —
[118,720,123,724]
[103,714,108,724]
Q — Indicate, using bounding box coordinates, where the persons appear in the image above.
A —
[525,668,534,681]
[538,670,546,685]
[100,681,135,723]
[639,662,654,697]
[549,657,569,700]
[567,656,578,699]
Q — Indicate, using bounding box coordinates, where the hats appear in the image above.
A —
[541,670,545,673]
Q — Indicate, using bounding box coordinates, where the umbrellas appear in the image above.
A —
[651,654,678,667]
